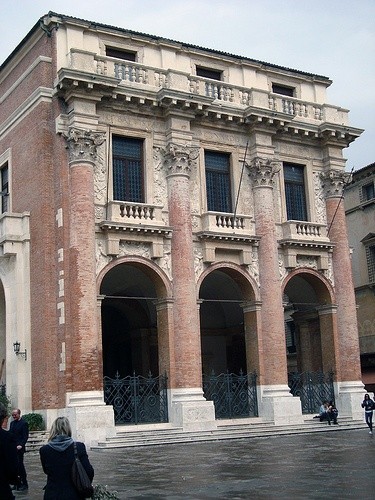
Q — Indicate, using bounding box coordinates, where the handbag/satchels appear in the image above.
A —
[71,442,93,498]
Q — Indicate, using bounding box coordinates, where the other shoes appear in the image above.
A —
[328,422,331,425]
[368,431,372,434]
[333,422,338,424]
[12,485,20,489]
[18,484,28,491]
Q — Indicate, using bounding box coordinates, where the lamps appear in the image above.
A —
[13,341,27,361]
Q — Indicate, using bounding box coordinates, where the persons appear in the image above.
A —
[361,394,375,434]
[9,408,31,490]
[0,409,22,500]
[39,417,94,500]
[319,400,339,425]
